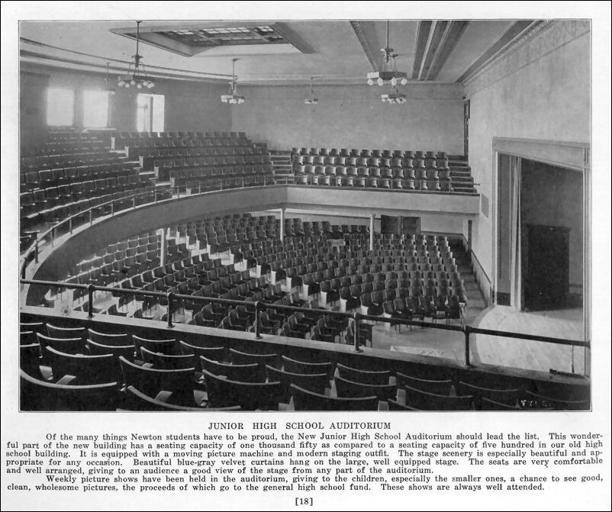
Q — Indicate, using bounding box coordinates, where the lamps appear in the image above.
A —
[221,58,245,105]
[366,21,407,86]
[377,86,407,107]
[303,77,319,105]
[118,21,153,90]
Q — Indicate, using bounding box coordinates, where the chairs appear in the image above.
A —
[20,128,591,410]
